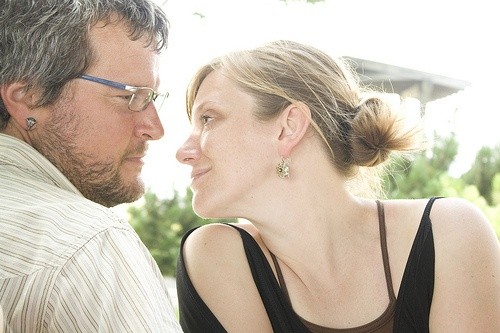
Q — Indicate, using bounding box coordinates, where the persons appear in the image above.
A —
[1,0,186,333]
[175,39,500,333]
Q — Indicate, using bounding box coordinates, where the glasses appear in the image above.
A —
[72,73,159,112]
